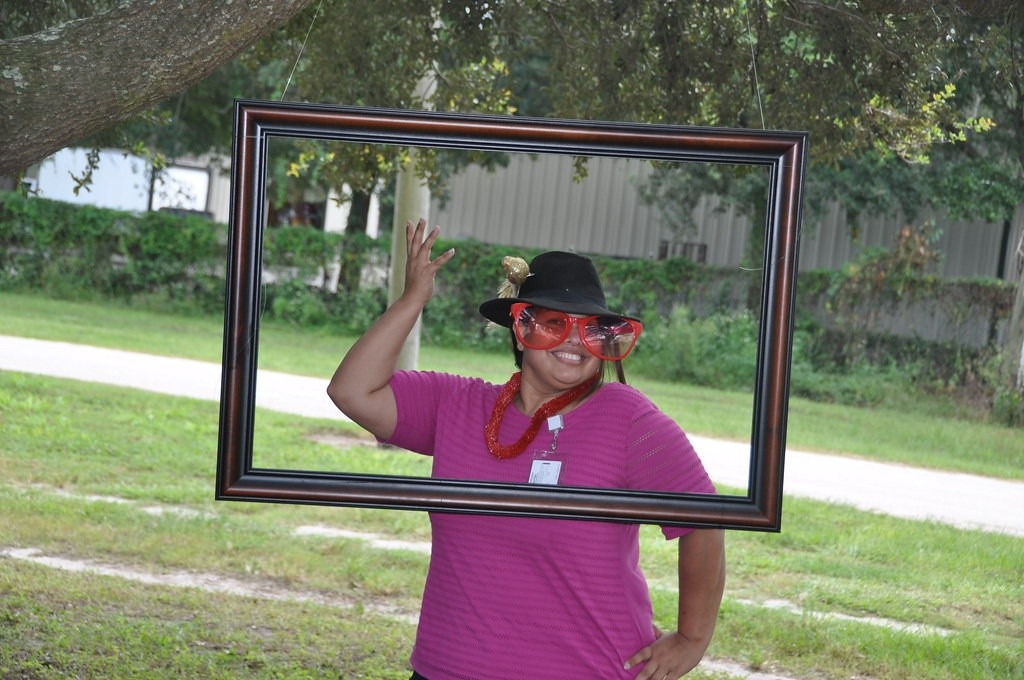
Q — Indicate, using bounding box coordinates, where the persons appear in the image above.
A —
[327,216,726,680]
[274,202,313,228]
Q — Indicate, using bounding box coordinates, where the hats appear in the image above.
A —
[478,250,641,329]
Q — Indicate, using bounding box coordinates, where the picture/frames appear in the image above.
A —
[213,96,807,535]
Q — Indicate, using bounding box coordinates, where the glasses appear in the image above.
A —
[508,301,643,361]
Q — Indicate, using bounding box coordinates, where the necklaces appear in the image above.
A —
[484,370,602,460]
